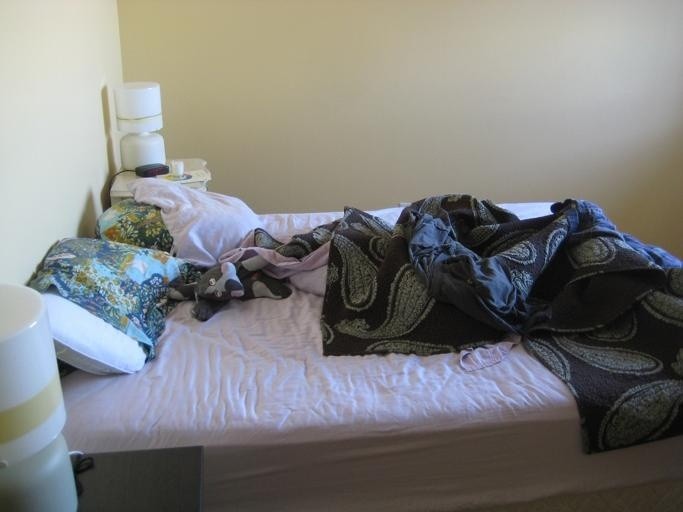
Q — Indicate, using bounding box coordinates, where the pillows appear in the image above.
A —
[27,186,180,376]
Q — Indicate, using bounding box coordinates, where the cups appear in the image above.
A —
[171,160,184,178]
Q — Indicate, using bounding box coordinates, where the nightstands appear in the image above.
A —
[70,445,203,512]
[111,160,210,206]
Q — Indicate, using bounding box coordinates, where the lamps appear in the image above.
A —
[0,282,80,512]
[113,80,167,170]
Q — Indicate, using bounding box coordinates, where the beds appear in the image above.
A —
[60,201,682,512]
